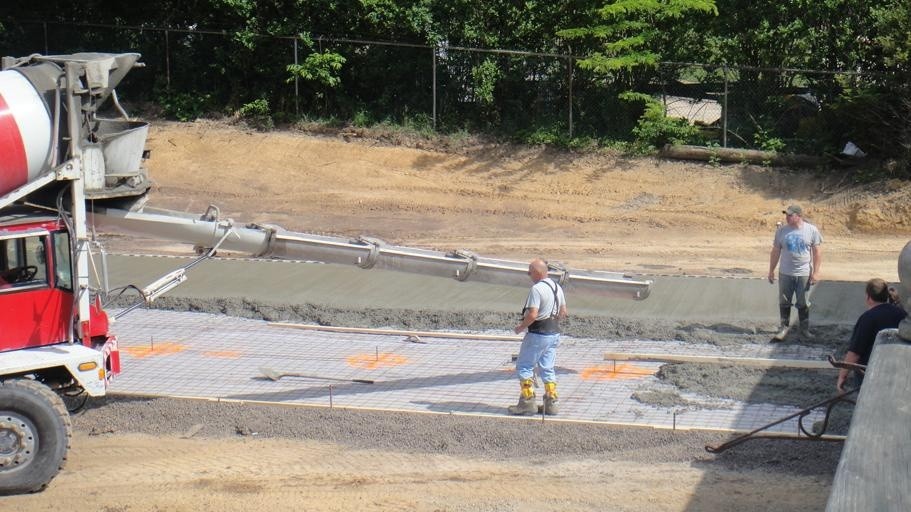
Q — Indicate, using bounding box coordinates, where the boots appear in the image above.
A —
[775,303,792,341]
[798,308,815,338]
[539,395,559,416]
[509,394,538,416]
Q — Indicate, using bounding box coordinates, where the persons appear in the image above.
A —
[508,259,568,417]
[768,205,824,342]
[836,278,911,397]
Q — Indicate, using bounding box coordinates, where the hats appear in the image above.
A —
[783,204,802,216]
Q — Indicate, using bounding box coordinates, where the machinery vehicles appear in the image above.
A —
[1,53,652,512]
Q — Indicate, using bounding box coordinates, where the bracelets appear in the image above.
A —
[892,299,901,306]
